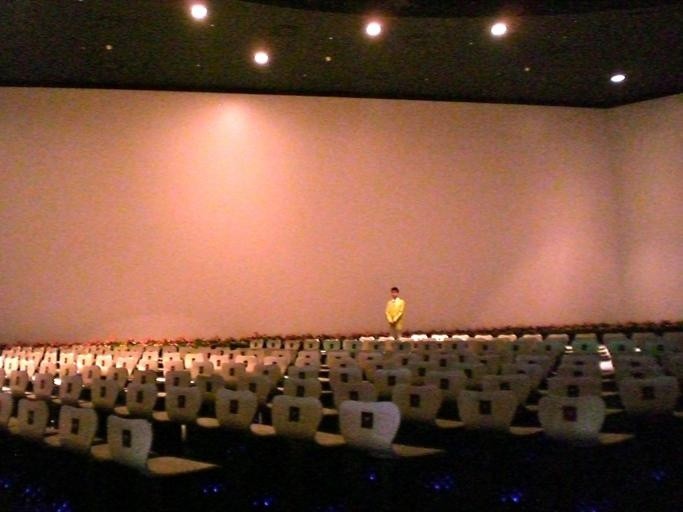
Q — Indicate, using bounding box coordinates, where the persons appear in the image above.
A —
[386,283,407,343]
